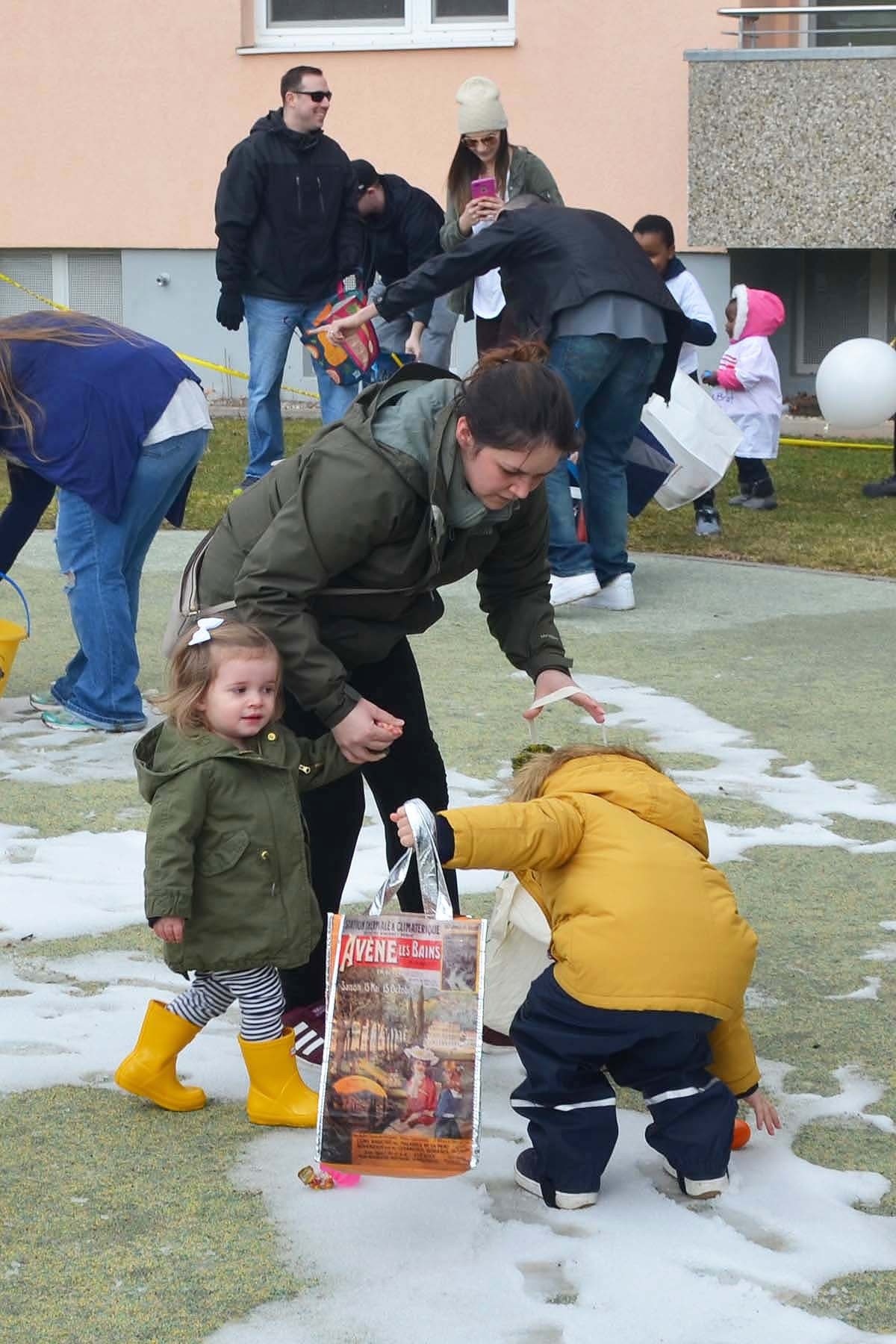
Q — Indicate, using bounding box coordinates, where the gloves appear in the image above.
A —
[216,287,245,331]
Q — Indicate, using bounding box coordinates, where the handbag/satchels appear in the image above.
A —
[283,277,380,385]
[638,366,743,511]
[160,534,227,659]
[314,798,487,1180]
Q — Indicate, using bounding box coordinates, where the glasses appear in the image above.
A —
[462,132,496,147]
[298,91,331,102]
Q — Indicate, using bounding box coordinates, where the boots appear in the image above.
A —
[238,1027,320,1128]
[114,999,206,1111]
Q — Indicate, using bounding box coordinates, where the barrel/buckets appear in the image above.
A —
[0,572,31,695]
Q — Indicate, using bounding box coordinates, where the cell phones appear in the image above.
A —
[472,176,497,218]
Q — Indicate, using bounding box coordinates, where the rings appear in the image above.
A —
[330,331,334,334]
[470,199,477,205]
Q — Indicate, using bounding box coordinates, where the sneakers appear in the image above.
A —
[570,573,635,611]
[41,708,102,731]
[694,507,720,537]
[29,692,65,711]
[547,572,601,607]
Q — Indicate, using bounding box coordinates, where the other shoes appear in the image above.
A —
[513,1146,598,1209]
[863,477,896,497]
[232,476,259,497]
[280,1001,326,1070]
[728,494,751,505]
[663,1157,730,1198]
[481,1025,517,1055]
[741,496,776,509]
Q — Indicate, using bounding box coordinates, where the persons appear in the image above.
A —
[631,214,722,536]
[0,313,214,734]
[178,336,609,1076]
[437,77,565,365]
[391,744,780,1209]
[349,158,461,373]
[114,613,402,1126]
[308,192,688,612]
[700,284,784,511]
[215,66,362,491]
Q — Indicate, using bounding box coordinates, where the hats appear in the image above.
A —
[455,77,508,134]
[350,159,377,198]
